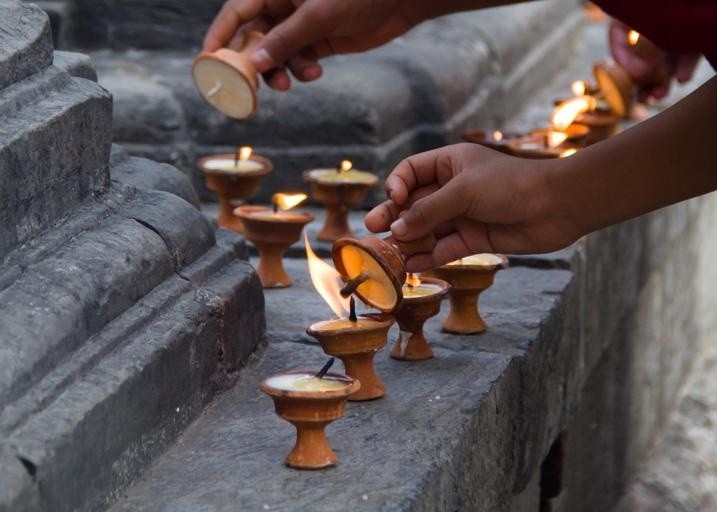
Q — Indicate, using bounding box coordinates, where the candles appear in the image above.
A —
[192,29,267,119]
[389,275,452,359]
[576,108,619,126]
[438,251,510,335]
[318,211,436,312]
[257,359,357,470]
[299,166,378,243]
[510,133,580,158]
[530,123,590,140]
[231,194,314,287]
[466,127,520,151]
[195,145,273,232]
[593,62,669,118]
[308,308,394,401]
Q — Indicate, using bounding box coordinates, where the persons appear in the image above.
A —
[199,1,537,93]
[365,1,717,273]
